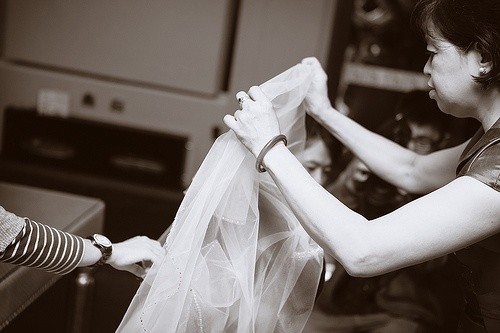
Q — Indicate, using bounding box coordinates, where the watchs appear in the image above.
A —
[85,233,112,270]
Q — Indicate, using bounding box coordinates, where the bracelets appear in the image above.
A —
[255,134,287,173]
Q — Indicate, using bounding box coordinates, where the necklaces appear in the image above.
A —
[132,282,205,333]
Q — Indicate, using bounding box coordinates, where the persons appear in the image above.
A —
[118,183,279,333]
[0,202,168,323]
[265,86,459,333]
[223,1,500,333]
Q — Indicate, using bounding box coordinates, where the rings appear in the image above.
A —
[238,95,249,109]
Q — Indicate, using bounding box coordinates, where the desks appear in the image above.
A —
[0,182,105,333]
